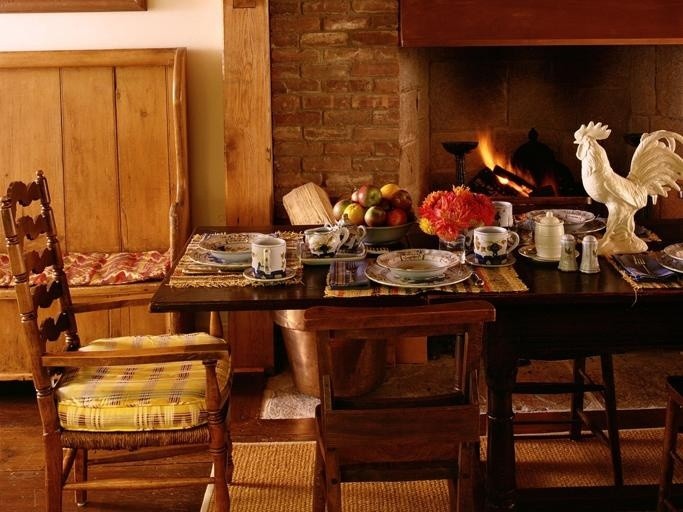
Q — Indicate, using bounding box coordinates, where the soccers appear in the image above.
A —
[333,183,412,227]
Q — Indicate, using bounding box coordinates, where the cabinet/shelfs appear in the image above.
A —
[303,214,367,258]
[535,210,600,273]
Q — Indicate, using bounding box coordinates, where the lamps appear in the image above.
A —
[296,300,497,512]
[0,168,238,511]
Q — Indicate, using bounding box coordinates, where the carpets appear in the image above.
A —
[259,346,680,422]
[200,424,681,512]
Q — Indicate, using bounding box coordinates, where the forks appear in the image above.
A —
[634,254,656,276]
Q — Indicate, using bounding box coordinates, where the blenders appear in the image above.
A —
[573,121,683,255]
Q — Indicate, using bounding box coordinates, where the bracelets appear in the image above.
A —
[367,218,417,245]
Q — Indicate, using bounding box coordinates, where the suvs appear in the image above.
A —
[271,308,388,399]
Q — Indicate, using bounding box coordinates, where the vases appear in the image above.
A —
[438,234,466,265]
[413,184,496,242]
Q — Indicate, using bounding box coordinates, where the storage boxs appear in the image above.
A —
[0,47,192,386]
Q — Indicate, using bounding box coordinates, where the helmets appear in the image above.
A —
[524,208,605,234]
[188,233,296,281]
[657,243,682,273]
[365,245,579,288]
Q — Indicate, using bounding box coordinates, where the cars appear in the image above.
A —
[472,200,520,265]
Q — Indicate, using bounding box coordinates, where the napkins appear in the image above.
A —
[612,249,678,284]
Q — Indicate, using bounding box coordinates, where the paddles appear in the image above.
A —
[200,424,681,512]
[259,346,680,422]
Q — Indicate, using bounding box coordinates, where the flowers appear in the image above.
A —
[413,184,496,242]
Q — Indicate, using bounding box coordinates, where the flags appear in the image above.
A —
[438,234,466,265]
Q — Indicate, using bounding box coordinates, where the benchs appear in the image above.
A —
[0,47,192,386]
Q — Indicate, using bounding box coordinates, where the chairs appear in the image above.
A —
[485,194,625,511]
[0,168,238,511]
[296,300,497,512]
[659,371,680,510]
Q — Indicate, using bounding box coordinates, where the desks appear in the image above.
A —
[148,216,681,512]
